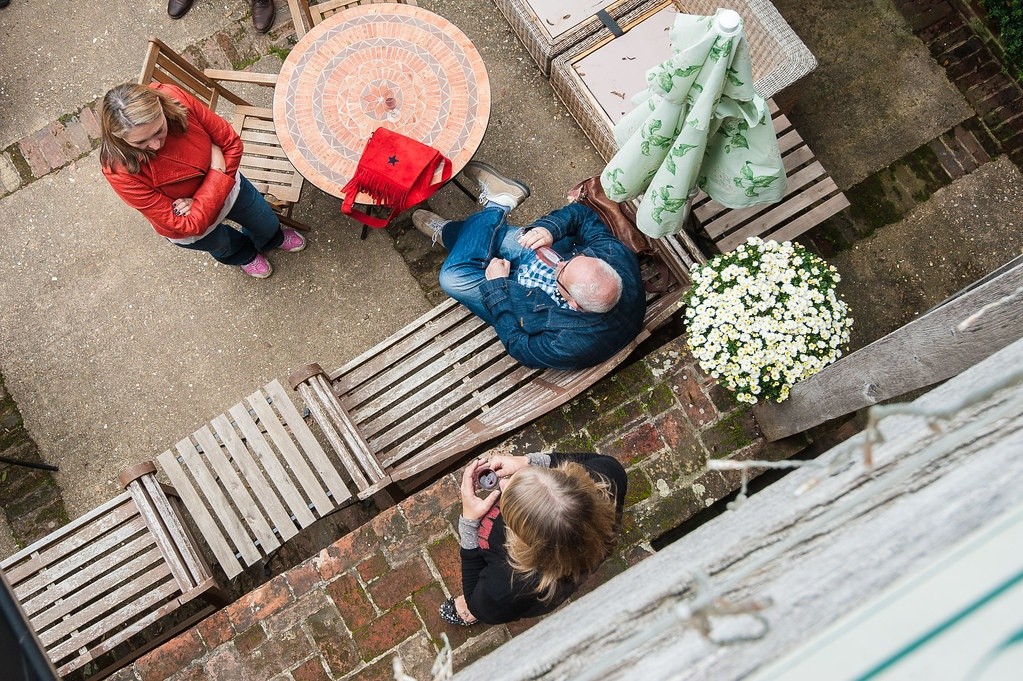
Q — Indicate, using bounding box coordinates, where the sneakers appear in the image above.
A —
[276,228,307,252]
[241,254,274,279]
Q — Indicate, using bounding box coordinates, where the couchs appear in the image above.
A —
[491,0,819,163]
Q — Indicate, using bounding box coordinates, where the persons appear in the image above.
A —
[438,451,629,627]
[99,82,307,279]
[412,160,647,371]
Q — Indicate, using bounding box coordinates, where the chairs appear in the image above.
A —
[138,35,313,232]
[288,0,419,40]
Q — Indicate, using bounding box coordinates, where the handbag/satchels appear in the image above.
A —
[342,127,453,229]
[567,175,668,293]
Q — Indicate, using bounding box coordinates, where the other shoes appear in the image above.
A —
[413,208,453,248]
[463,160,530,211]
[438,597,478,625]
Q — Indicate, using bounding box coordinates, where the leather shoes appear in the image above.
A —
[168,0,194,19]
[251,0,276,32]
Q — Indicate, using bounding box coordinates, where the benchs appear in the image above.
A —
[0,460,229,681]
[284,191,712,494]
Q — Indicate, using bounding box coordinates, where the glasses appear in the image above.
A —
[556,250,586,310]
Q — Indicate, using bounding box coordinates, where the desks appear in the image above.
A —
[272,3,491,205]
[694,97,852,256]
[163,378,374,582]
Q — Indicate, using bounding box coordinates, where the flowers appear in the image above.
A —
[680,235,856,404]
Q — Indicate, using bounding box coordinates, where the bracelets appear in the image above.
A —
[210,165,225,174]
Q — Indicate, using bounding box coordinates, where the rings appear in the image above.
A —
[533,230,538,234]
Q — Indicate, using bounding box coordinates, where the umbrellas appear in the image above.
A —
[600,9,789,240]
